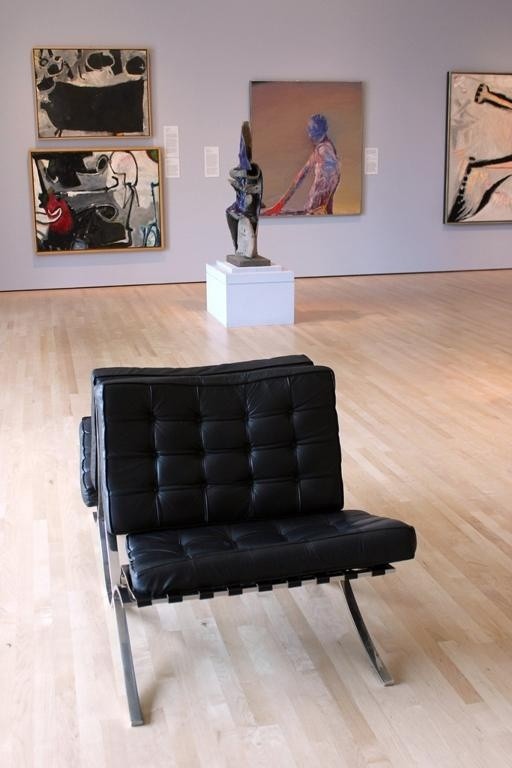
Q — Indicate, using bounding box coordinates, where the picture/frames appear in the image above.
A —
[442,66,512,224]
[26,44,170,258]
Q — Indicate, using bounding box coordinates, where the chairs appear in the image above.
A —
[80,353,418,727]
